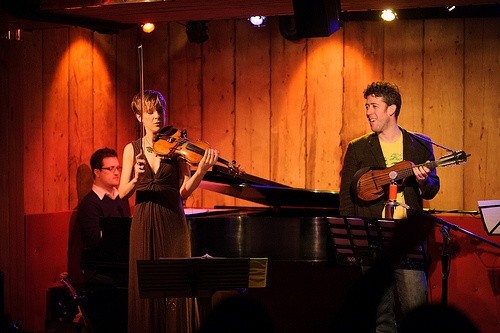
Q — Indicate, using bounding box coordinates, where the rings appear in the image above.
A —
[137,161,140,165]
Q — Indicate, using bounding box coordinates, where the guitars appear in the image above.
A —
[350,150,468,207]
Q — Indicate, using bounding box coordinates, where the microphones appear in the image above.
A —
[389,171,398,202]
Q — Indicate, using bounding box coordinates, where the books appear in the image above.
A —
[477,200,500,234]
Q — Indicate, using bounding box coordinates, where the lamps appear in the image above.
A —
[446,4,456,11]
[378,8,398,23]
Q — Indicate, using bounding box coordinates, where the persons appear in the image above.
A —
[346,210,480,333]
[76,148,133,286]
[118,89,220,333]
[339,81,440,333]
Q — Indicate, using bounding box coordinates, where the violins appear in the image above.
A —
[153,126,245,178]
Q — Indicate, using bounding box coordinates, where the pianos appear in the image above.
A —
[81,160,340,280]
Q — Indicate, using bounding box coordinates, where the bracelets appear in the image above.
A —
[132,178,141,183]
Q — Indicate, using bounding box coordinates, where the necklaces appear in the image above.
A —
[145,139,153,153]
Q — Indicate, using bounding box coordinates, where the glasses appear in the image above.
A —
[100,165,122,171]
